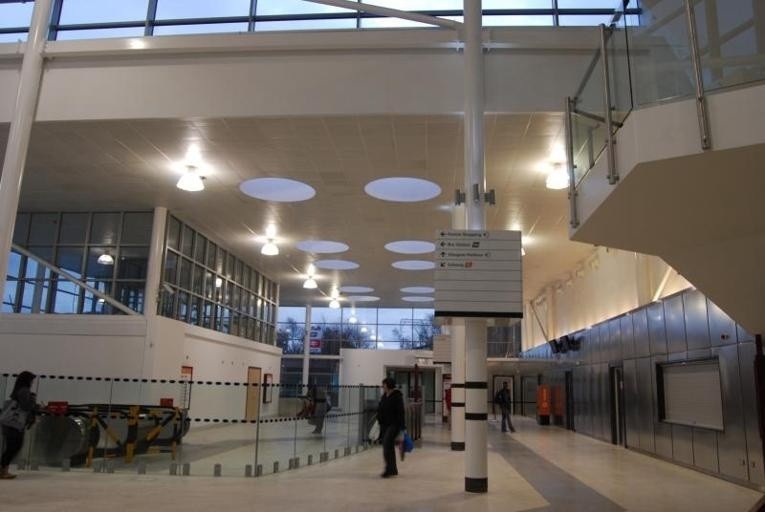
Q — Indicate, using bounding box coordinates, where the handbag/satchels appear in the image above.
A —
[399,440,404,461]
[368,416,380,440]
[2,400,28,430]
[404,433,413,452]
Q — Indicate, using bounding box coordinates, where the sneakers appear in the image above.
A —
[382,470,392,478]
[391,469,398,475]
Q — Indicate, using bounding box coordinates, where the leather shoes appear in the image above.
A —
[1,473,17,479]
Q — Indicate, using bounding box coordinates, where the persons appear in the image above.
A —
[494,381,516,433]
[307,383,328,434]
[0,370,38,479]
[376,378,406,479]
